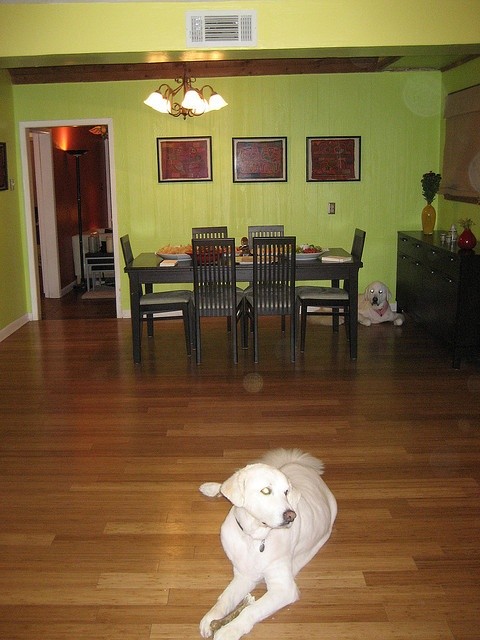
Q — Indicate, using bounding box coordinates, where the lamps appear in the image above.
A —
[143,62,229,121]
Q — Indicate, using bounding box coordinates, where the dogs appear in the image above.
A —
[306,281,405,327]
[198,446,339,640]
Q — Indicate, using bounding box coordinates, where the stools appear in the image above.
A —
[91,265,114,292]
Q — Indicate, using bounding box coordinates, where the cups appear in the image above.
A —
[440,234,446,241]
[446,236,451,243]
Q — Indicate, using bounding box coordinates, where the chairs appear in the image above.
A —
[244,237,295,363]
[244,225,295,333]
[191,238,245,365]
[192,226,244,334]
[295,228,366,359]
[120,233,196,357]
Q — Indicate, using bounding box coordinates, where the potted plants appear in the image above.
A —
[420,171,442,235]
[457,217,477,250]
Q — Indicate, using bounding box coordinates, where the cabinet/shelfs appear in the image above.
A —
[396,230,480,370]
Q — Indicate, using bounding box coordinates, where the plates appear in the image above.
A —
[155,251,193,261]
[220,256,280,264]
[282,250,330,261]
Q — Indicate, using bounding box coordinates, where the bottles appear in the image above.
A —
[106,236,113,254]
[88,237,97,253]
[90,231,99,252]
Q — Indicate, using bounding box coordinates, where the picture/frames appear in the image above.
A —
[0,142,8,191]
[305,136,361,182]
[232,136,288,183]
[156,135,213,183]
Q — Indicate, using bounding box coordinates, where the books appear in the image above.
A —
[159,259,179,267]
[321,255,353,263]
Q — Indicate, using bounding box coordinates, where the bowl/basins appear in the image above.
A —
[185,249,228,265]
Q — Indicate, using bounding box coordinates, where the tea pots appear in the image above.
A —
[448,224,458,241]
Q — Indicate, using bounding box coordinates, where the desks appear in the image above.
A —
[85,250,114,292]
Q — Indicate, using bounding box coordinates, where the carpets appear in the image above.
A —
[81,283,115,299]
